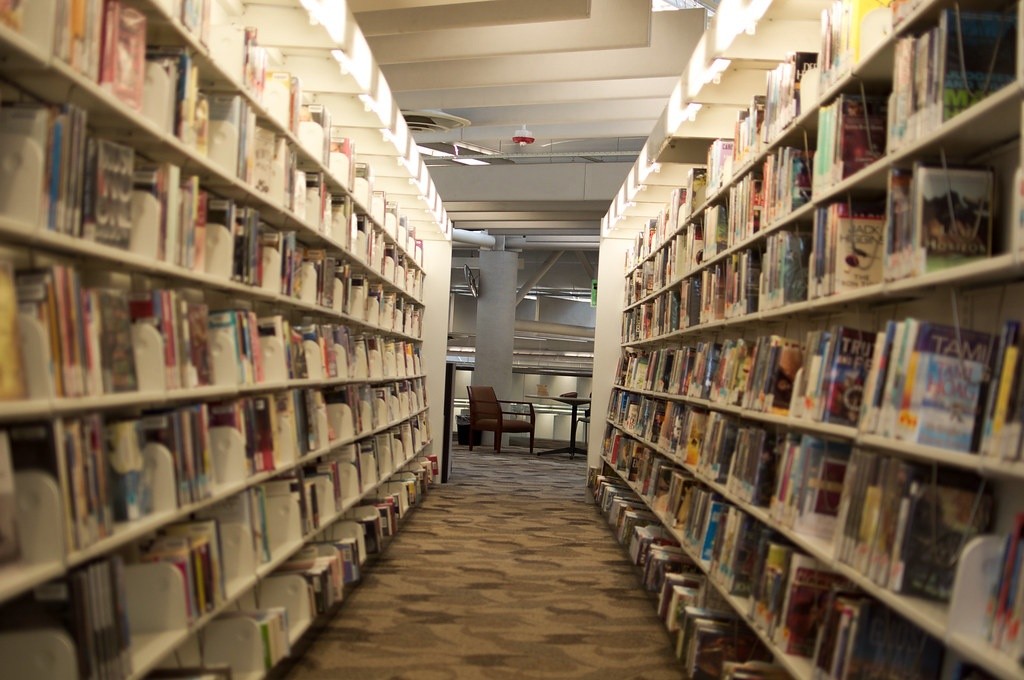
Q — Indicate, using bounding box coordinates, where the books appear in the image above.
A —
[0,0,439,680]
[586,0,1024,680]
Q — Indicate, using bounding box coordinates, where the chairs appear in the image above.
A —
[576,392,592,446]
[467,386,535,453]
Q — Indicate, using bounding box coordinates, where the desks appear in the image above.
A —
[526,395,591,460]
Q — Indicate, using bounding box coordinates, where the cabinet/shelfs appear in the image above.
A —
[0,1,433,679]
[600,0,1024,680]
